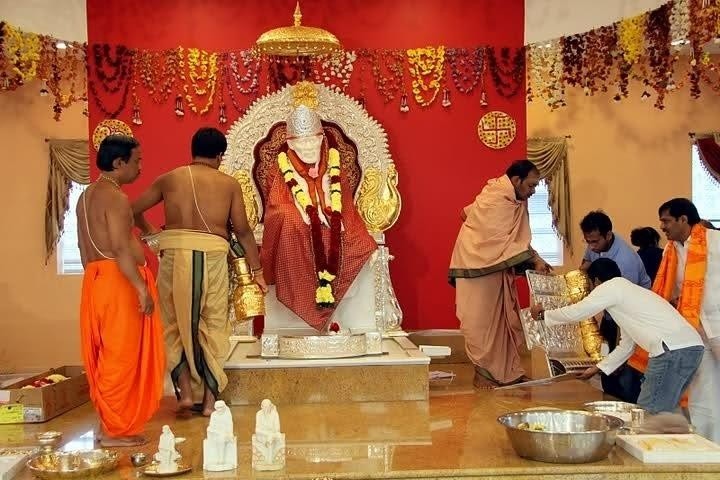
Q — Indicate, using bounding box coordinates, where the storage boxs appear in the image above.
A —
[0,365,90,424]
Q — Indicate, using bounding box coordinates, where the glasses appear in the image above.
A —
[579,237,603,246]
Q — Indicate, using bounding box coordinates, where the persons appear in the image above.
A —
[530,257,706,414]
[630,227,665,286]
[448,158,553,388]
[652,198,720,446]
[76,134,167,447]
[130,127,268,417]
[578,210,650,398]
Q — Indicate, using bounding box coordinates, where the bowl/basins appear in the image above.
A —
[497,408,626,464]
